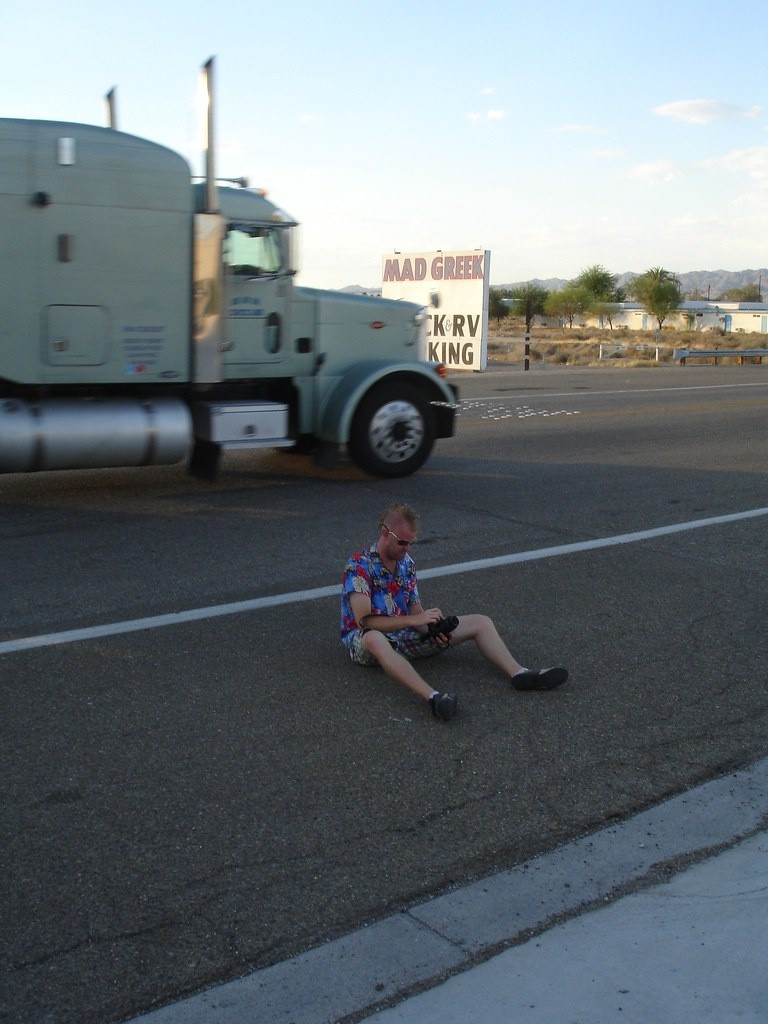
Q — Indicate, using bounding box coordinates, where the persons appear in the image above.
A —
[339,500,569,720]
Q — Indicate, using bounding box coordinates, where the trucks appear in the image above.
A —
[0,57,457,478]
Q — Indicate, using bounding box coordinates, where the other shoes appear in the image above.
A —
[428,691,457,720]
[511,667,568,691]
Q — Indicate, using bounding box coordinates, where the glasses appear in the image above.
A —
[382,523,416,546]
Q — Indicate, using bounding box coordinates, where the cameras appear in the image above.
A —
[427,615,459,640]
